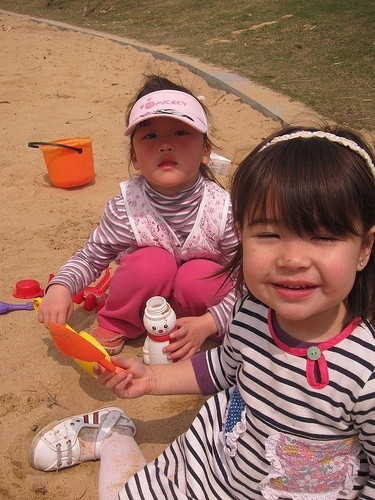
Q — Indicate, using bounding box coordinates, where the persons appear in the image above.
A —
[38,73,241,359]
[27,113,375,500]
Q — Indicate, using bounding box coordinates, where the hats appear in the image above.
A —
[124,89,208,139]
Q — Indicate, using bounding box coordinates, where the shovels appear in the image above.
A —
[33,297,128,375]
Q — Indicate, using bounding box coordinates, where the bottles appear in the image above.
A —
[140,293,179,368]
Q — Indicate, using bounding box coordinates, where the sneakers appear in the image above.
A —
[29,406,137,471]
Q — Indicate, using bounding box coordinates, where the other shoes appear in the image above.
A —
[91,324,127,355]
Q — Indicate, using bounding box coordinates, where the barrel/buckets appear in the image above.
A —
[25,133,97,190]
